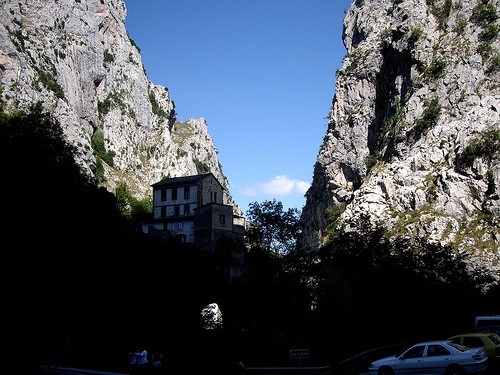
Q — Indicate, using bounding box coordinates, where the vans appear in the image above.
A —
[470,314,500,334]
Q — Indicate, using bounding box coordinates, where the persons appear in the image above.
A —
[127,343,164,371]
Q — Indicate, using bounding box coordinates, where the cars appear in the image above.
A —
[369,340,490,375]
[446,330,500,366]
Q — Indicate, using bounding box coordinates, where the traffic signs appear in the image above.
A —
[289,348,310,368]
